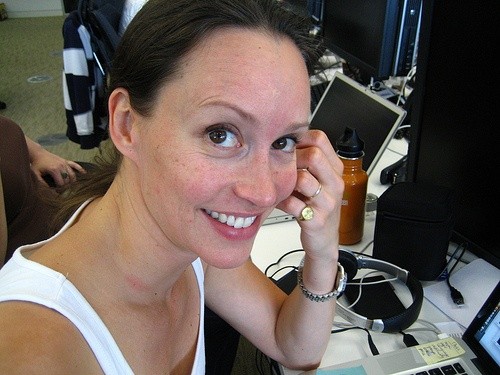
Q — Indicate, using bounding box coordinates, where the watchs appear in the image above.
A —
[296,254,347,303]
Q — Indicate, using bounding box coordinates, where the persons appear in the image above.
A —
[0,1,348,375]
[1,115,103,272]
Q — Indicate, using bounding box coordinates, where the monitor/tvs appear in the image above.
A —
[408,1,499,269]
[318,0,399,78]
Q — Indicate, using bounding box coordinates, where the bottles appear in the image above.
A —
[334,126,368,245]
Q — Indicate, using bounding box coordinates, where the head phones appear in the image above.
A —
[302,248,425,333]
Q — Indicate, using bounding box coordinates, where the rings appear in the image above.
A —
[61,170,70,179]
[306,182,322,200]
[295,201,315,222]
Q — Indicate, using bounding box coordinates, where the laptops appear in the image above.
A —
[292,277,500,374]
[262,70,407,226]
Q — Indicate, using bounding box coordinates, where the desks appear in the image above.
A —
[249,47,500,375]
